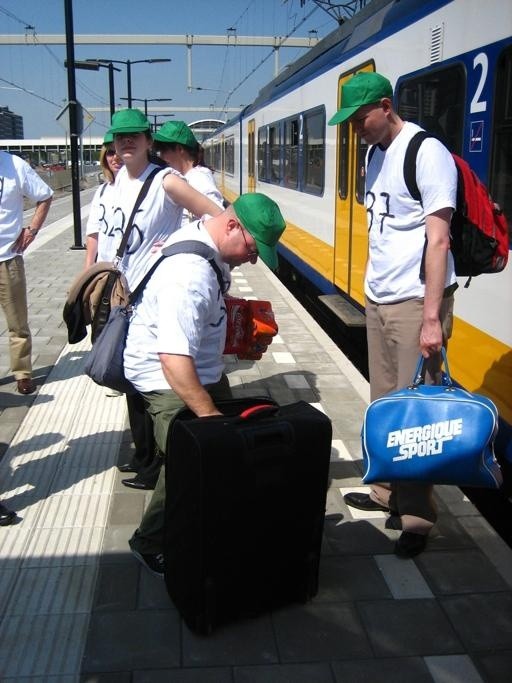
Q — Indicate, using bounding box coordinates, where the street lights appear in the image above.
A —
[84,56,173,109]
[61,57,115,126]
[118,94,177,133]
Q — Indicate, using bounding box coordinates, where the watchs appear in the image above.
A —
[27,226,38,235]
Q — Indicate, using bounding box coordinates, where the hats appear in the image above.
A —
[234,193,286,271]
[151,121,195,146]
[328,71,394,126]
[103,109,150,144]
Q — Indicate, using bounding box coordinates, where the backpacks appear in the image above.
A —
[368,131,509,276]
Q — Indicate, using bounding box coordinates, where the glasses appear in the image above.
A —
[238,223,258,256]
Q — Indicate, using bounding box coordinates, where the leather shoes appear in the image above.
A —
[344,492,388,511]
[118,462,138,472]
[121,478,152,490]
[18,379,34,394]
[395,531,428,559]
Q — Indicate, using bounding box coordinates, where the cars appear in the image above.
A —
[33,157,101,172]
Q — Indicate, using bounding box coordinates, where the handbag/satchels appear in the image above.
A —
[362,343,505,489]
[83,306,137,395]
[91,306,110,344]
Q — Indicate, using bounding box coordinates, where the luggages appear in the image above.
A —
[163,394,332,637]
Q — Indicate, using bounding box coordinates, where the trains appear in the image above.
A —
[200,0,512,498]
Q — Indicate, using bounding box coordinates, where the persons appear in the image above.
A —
[328,70,460,560]
[86,110,223,489]
[0,150,54,395]
[126,192,285,577]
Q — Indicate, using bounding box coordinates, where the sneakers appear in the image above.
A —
[133,550,166,576]
